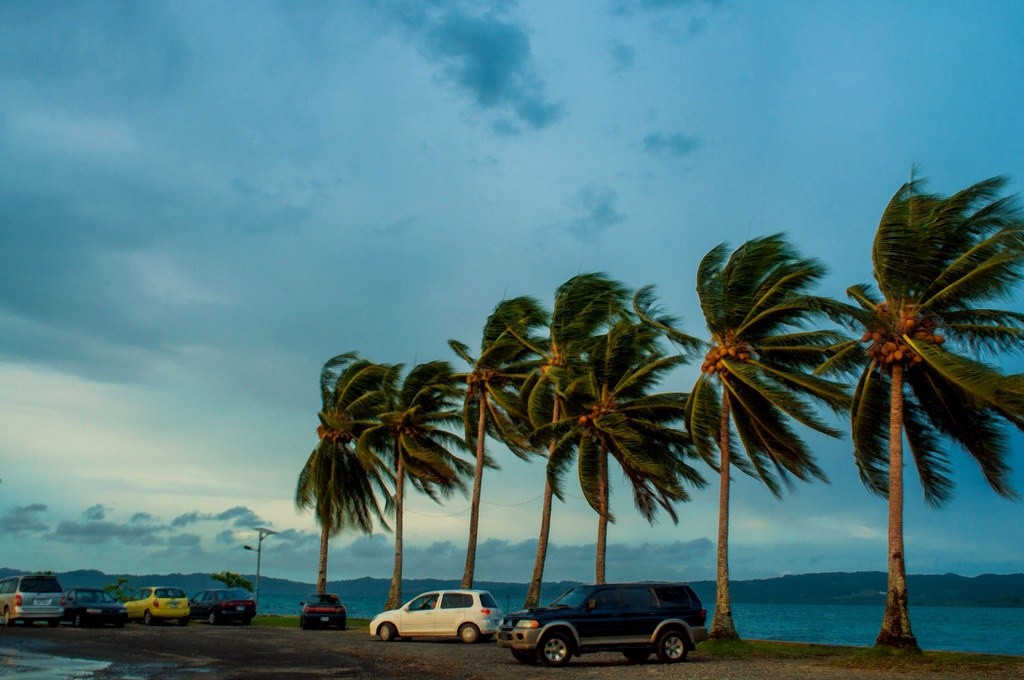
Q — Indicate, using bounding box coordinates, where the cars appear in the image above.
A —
[299,592,347,630]
[369,587,502,645]
[187,588,257,626]
[60,586,128,628]
[122,585,191,627]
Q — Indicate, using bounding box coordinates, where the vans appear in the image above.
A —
[0,574,66,628]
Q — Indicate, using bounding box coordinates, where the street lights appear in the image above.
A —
[244,527,279,618]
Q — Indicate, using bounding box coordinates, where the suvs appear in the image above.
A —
[495,580,708,667]
